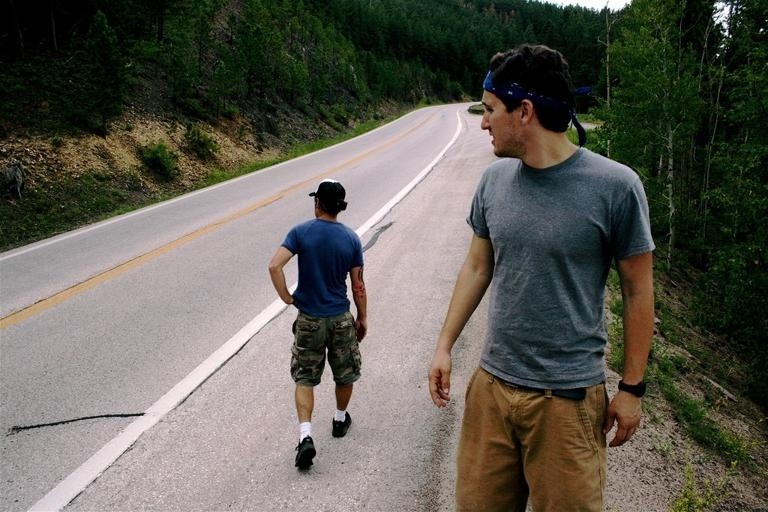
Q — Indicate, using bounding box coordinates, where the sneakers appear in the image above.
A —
[295,435,316,469]
[332,410,352,437]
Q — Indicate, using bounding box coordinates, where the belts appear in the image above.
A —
[501,379,588,400]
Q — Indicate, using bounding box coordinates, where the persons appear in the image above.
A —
[428,44,655,512]
[269,182,367,467]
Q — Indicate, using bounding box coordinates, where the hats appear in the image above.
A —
[308,179,348,210]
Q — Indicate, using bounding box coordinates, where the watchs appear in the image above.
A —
[618,379,647,398]
